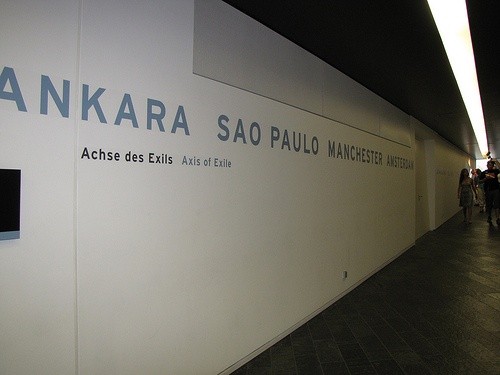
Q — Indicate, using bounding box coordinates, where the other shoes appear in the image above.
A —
[468,218,473,224]
[487,216,491,223]
[463,216,467,221]
[496,219,500,224]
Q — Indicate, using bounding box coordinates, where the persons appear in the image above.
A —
[480,158,500,224]
[474,169,485,214]
[458,168,478,224]
